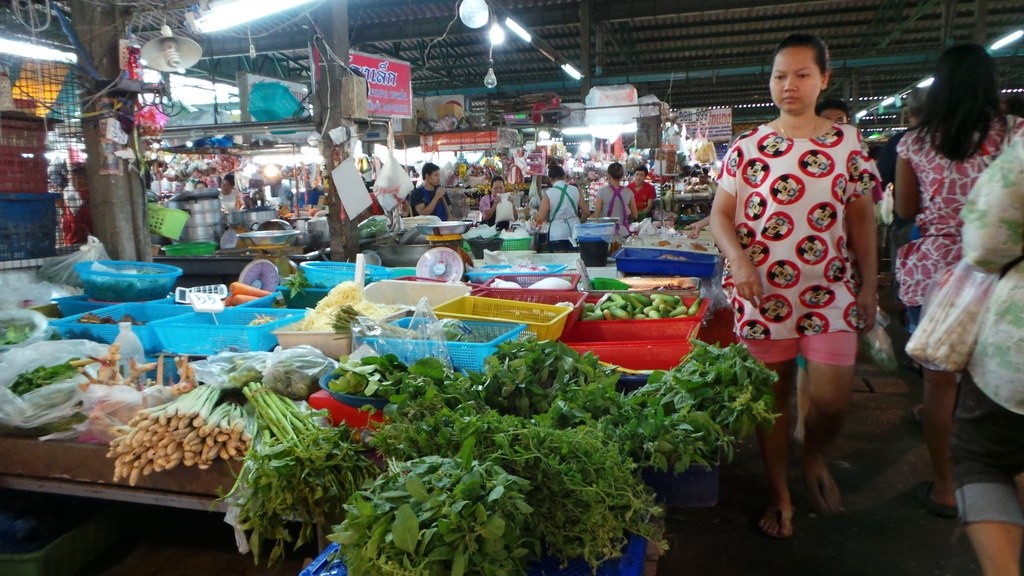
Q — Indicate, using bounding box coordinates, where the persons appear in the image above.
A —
[479,175,518,231]
[625,165,657,225]
[680,31,884,536]
[47,167,95,249]
[536,159,590,251]
[590,161,638,241]
[409,162,452,221]
[870,41,1024,576]
[194,168,245,213]
[297,178,326,207]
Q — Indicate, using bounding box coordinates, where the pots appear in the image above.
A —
[250,219,293,231]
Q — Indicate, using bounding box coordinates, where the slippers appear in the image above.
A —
[916,481,957,517]
[758,491,794,539]
[794,450,841,517]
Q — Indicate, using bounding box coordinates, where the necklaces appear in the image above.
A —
[778,116,817,139]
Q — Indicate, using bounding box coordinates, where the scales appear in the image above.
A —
[236,229,302,291]
[416,221,473,283]
[587,217,620,256]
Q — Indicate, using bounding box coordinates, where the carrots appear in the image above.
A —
[223,282,273,306]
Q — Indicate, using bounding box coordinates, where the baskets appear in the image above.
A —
[50,201,725,410]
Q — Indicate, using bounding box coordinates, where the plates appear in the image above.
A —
[318,361,470,410]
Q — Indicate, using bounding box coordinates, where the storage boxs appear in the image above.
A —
[638,462,720,506]
[0,503,126,576]
[300,534,650,576]
[49,247,724,381]
[0,118,57,259]
[437,103,463,122]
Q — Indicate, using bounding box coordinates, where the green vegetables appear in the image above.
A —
[284,269,314,299]
[0,304,785,576]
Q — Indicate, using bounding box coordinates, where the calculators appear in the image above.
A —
[174,284,227,304]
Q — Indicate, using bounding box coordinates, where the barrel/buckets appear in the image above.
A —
[577,235,609,267]
[467,237,504,260]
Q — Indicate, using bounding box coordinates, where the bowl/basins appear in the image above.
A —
[377,243,448,268]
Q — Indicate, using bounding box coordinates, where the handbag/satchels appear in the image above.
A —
[860,306,896,375]
[905,254,1001,373]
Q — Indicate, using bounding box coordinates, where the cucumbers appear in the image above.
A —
[577,292,702,320]
